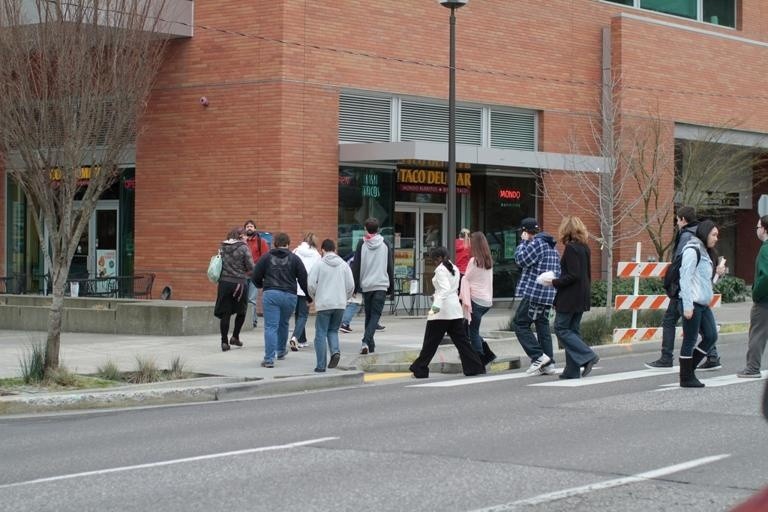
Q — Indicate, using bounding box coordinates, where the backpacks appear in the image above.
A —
[662,246,700,300]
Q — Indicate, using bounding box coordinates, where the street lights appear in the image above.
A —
[436,0,468,266]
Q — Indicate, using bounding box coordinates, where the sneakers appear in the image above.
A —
[736,367,761,378]
[222,343,230,351]
[526,352,555,376]
[360,342,369,354]
[696,355,721,371]
[230,336,242,346]
[289,336,308,351]
[314,368,325,372]
[276,349,288,360]
[644,355,674,369]
[260,360,275,368]
[340,322,385,333]
[327,353,340,368]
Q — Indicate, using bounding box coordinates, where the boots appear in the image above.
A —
[679,348,706,387]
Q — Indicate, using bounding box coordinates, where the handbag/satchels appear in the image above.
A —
[207,255,222,283]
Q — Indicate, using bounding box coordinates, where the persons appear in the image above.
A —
[408,214,599,382]
[644,206,723,370]
[211,215,394,372]
[737,214,768,378]
[676,218,725,388]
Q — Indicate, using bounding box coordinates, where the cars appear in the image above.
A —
[485,230,510,265]
[337,222,393,261]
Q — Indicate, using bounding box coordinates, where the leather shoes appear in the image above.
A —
[582,354,599,377]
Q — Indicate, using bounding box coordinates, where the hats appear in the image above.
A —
[516,217,539,231]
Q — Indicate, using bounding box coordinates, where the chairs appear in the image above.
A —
[389,275,420,315]
[0,273,157,299]
